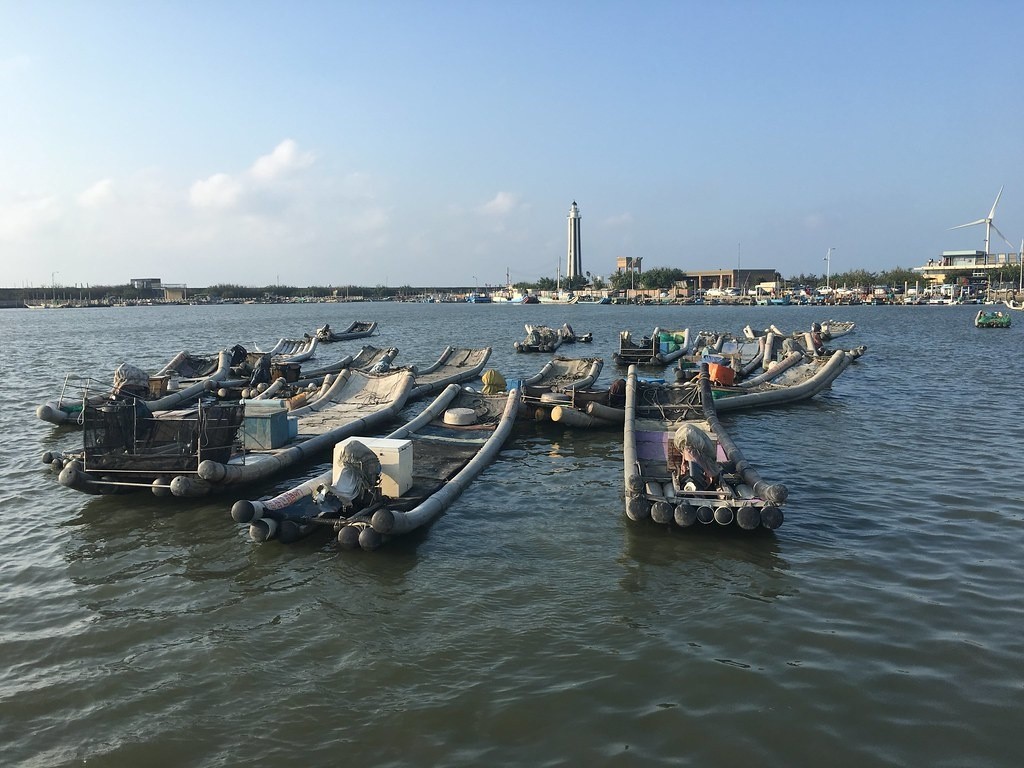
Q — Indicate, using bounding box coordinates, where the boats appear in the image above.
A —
[464,266,612,305]
[523,350,852,429]
[513,322,594,353]
[57,369,415,498]
[230,383,522,552]
[622,362,790,531]
[612,318,857,381]
[975,310,1012,328]
[37,320,493,425]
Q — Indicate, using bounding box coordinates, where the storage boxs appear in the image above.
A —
[236,406,289,450]
[667,431,718,470]
[287,416,298,439]
[333,435,414,497]
[149,375,171,396]
